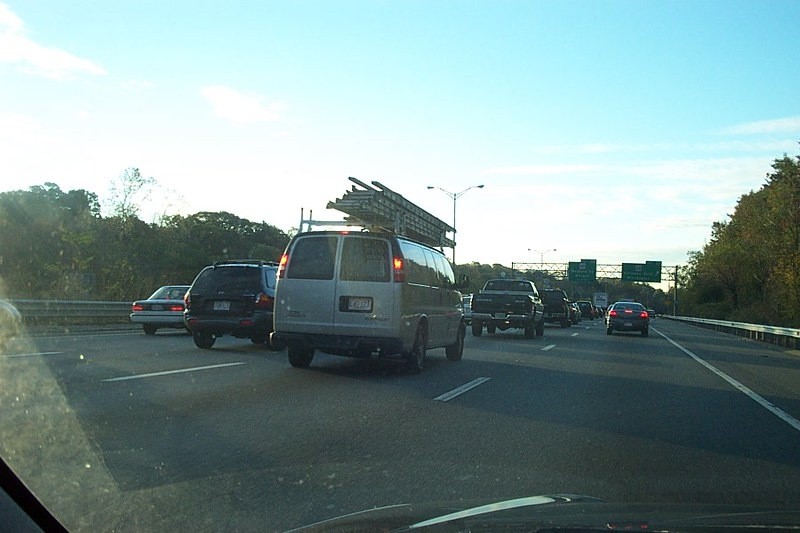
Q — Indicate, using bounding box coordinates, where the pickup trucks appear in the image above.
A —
[537,289,572,329]
[469,278,546,340]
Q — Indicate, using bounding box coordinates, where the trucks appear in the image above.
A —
[592,290,608,311]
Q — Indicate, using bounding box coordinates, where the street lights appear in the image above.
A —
[427,184,485,272]
[528,248,558,263]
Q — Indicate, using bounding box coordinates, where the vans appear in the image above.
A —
[268,207,471,375]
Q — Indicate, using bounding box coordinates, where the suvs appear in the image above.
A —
[462,295,474,318]
[182,259,280,352]
[577,300,596,320]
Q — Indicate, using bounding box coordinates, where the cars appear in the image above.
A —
[593,305,607,318]
[604,304,627,325]
[647,310,655,319]
[571,303,582,325]
[606,302,649,337]
[129,285,190,335]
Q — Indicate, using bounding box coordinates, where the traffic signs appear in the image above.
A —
[620,260,661,282]
[567,259,596,283]
